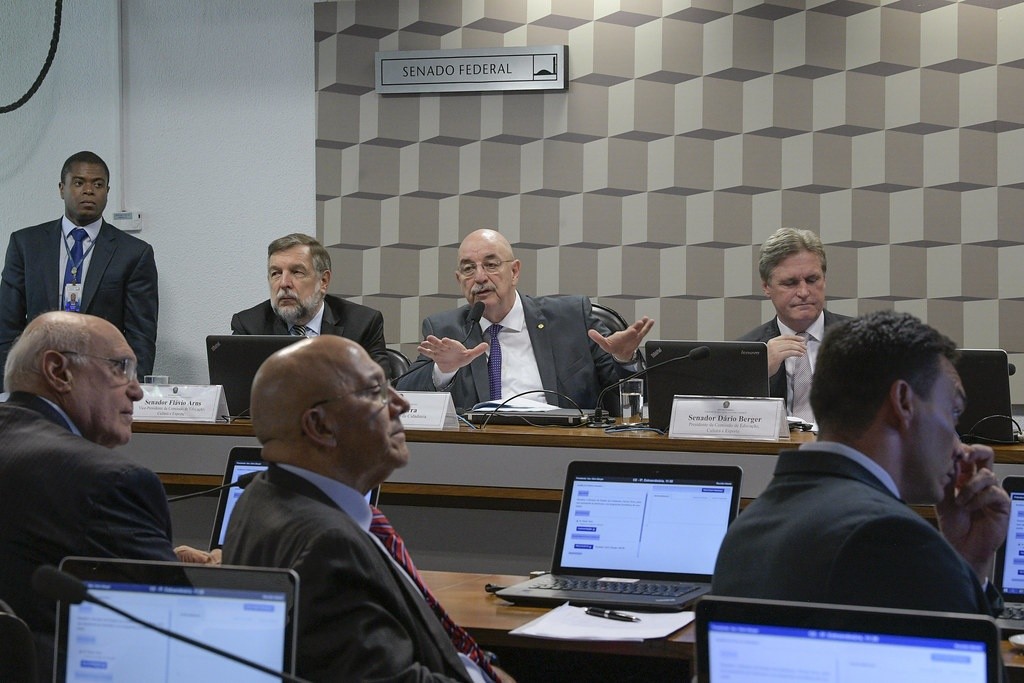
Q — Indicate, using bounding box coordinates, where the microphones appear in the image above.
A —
[30,564,311,683]
[587,346,710,428]
[390,301,484,385]
[167,470,262,503]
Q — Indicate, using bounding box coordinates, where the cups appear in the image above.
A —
[143,375,170,385]
[618,379,644,426]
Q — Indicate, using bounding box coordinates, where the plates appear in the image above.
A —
[1007,633,1024,652]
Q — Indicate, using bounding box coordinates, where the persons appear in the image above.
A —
[230,233,391,383]
[730,228,856,424]
[396,229,654,416]
[0,151,159,383]
[714,309,1012,616]
[65,293,79,310]
[222,335,516,683]
[0,309,222,683]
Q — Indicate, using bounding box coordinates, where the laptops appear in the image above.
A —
[644,340,802,432]
[208,446,380,554]
[951,348,1022,444]
[53,556,300,683]
[693,594,1000,683]
[993,475,1024,634]
[206,334,308,418]
[462,408,610,425]
[497,460,743,612]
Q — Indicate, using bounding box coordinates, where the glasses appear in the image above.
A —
[458,257,515,277]
[61,348,138,384]
[300,378,393,437]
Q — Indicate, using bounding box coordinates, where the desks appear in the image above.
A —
[189,538,1024,683]
[99,390,811,494]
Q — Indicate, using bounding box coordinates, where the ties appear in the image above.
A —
[293,322,308,336]
[62,228,89,312]
[791,330,815,424]
[372,504,502,683]
[487,323,506,401]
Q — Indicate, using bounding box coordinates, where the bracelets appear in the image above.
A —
[613,350,636,362]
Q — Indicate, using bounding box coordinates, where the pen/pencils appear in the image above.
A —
[585,607,642,622]
